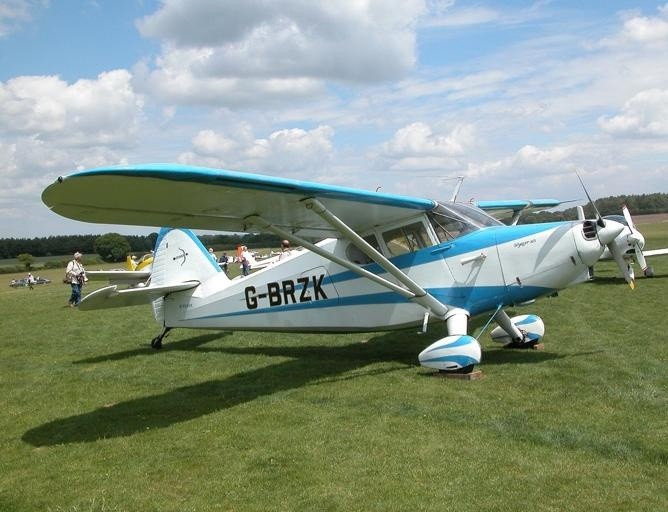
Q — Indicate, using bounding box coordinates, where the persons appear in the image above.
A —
[64,250,89,308]
[26,272,35,291]
[276,239,292,262]
[129,255,138,270]
[241,256,251,277]
[218,250,229,276]
[207,247,217,262]
[238,245,249,275]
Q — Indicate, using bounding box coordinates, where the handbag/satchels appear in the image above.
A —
[63,271,72,284]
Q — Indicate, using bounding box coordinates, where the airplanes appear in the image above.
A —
[82,248,230,288]
[42,164,636,380]
